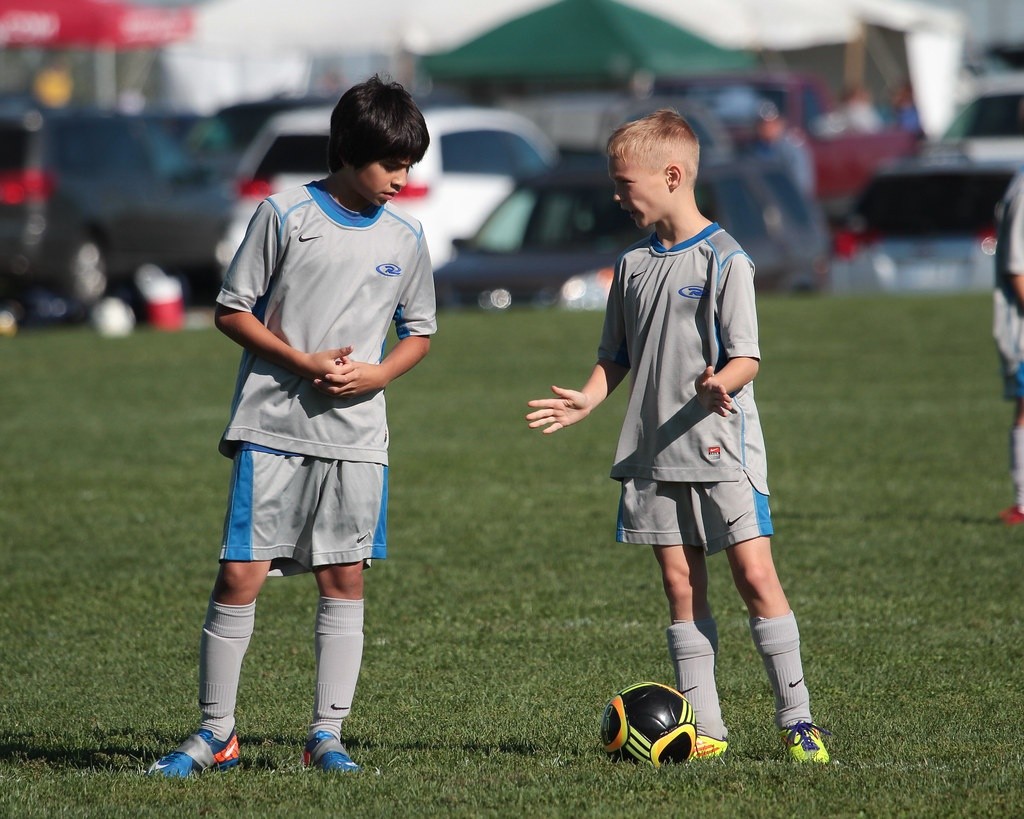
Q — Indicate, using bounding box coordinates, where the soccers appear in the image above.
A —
[601,681,698,767]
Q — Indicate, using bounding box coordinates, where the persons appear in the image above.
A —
[527,107,831,767]
[993,166,1024,521]
[143,78,439,779]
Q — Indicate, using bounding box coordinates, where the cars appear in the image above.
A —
[642,71,910,230]
[214,103,570,302]
[424,145,828,309]
[0,96,232,311]
[821,138,1023,291]
[918,73,1024,163]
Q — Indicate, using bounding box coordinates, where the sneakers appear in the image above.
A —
[145,726,242,779]
[301,731,362,776]
[778,724,828,765]
[998,504,1024,526]
[691,734,727,761]
